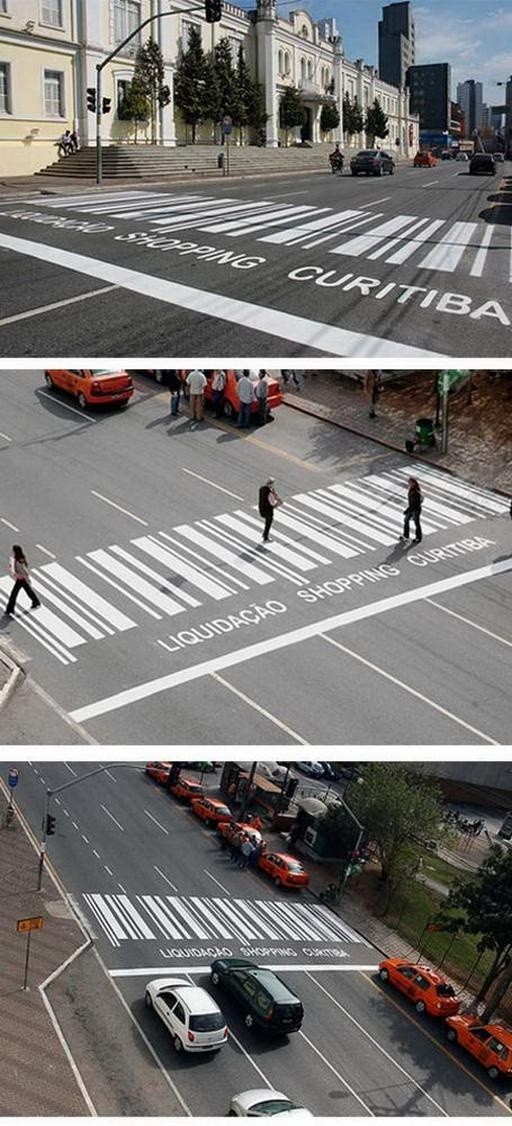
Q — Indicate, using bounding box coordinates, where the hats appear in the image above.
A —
[266,476,275,484]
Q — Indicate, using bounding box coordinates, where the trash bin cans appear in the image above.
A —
[217,152,225,176]
[415,418,434,447]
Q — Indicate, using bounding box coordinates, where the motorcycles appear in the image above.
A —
[331,155,344,173]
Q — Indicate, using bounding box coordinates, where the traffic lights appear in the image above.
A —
[204,0,222,22]
[102,96,111,113]
[47,814,56,835]
[86,87,95,113]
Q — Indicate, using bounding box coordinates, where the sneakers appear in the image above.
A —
[264,536,274,543]
[171,408,267,429]
[369,411,377,419]
[399,535,422,543]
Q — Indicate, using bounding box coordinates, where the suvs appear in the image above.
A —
[445,1013,512,1081]
[211,957,305,1039]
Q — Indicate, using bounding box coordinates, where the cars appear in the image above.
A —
[181,368,283,418]
[147,762,364,891]
[350,148,394,176]
[42,369,134,409]
[146,976,230,1053]
[145,369,192,385]
[230,1086,316,1116]
[413,147,506,176]
[378,954,459,1015]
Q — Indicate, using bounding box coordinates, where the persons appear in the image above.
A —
[3,545,40,616]
[399,477,423,544]
[331,148,344,167]
[58,129,78,155]
[165,369,269,428]
[230,830,266,870]
[281,369,299,384]
[258,476,276,543]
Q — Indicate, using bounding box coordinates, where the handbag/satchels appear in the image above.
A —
[416,489,424,503]
